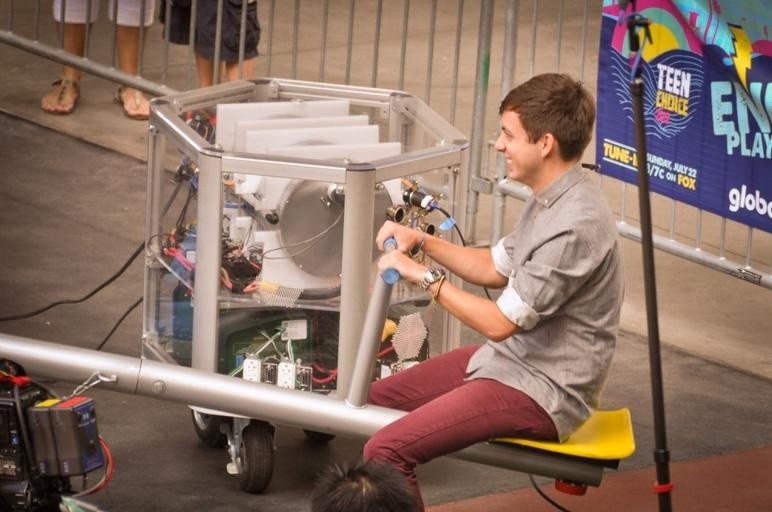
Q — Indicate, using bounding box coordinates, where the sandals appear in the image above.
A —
[40,71,80,115]
[115,78,153,121]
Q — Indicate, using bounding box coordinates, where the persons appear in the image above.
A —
[311,462,412,510]
[362,72,625,511]
[42,1,157,117]
[159,0,260,87]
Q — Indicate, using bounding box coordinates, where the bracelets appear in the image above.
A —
[416,231,425,251]
[429,276,446,310]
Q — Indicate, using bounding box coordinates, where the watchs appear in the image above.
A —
[420,268,447,294]
